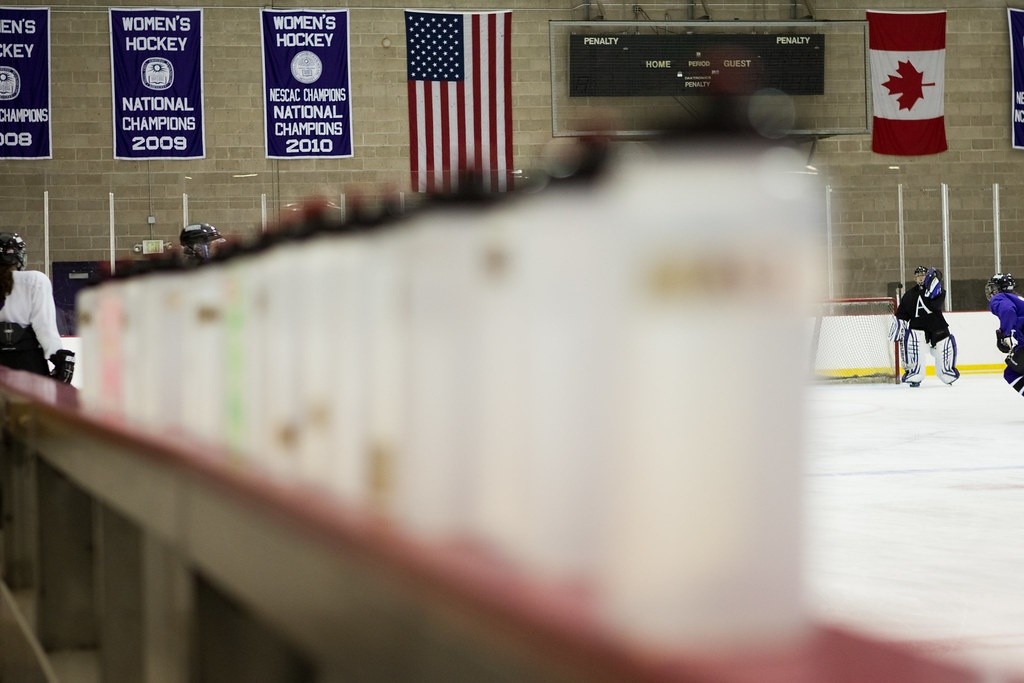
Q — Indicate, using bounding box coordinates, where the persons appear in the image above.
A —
[888,266,960,387]
[985,272,1024,397]
[0,231,76,385]
[179,222,227,265]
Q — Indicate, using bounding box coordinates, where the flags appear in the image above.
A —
[865,9,949,157]
[404,10,515,195]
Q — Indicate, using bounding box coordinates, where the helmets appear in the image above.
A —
[915,266,928,277]
[179,223,224,246]
[984,272,1015,302]
[0,232,28,270]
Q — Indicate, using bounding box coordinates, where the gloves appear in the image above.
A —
[48,349,76,385]
[996,329,1013,353]
